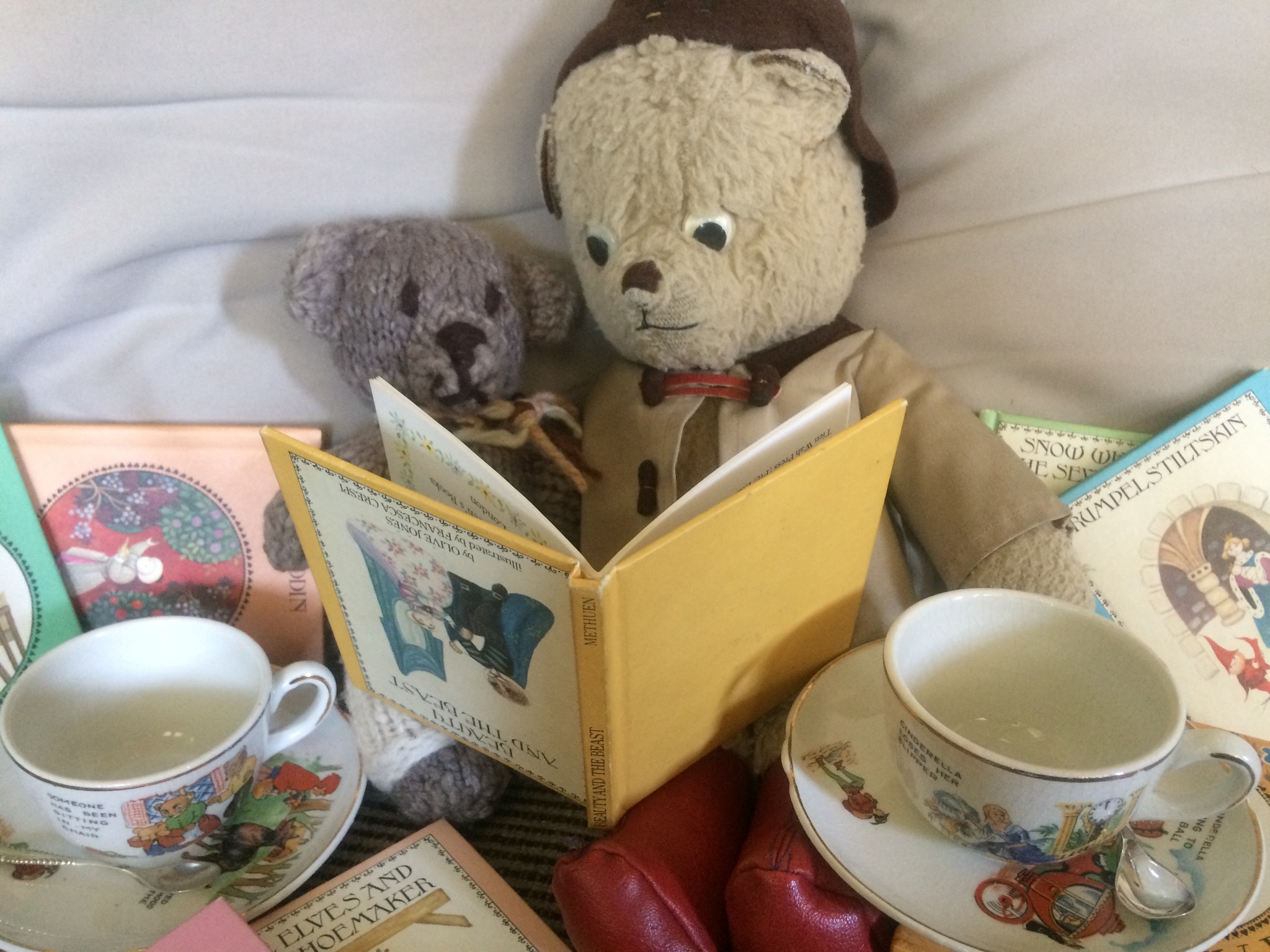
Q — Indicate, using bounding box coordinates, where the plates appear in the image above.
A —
[781,637,1264,951]
[0,662,368,952]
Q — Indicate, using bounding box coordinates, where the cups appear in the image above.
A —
[0,615,337,869]
[884,586,1263,866]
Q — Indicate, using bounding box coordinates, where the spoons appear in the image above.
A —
[0,843,221,894]
[1113,824,1196,922]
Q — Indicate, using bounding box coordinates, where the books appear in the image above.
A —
[0,423,324,707]
[142,896,272,952]
[888,368,1270,952]
[259,375,908,829]
[249,815,570,952]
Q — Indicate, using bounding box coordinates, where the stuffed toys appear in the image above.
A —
[263,214,603,822]
[538,0,1095,952]
